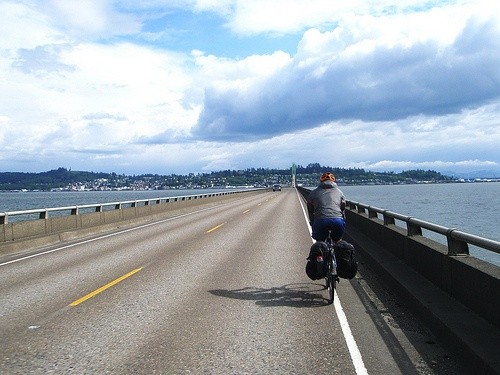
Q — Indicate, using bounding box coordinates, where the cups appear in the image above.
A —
[317,256,323,262]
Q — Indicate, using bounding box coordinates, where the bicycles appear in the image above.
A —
[323,227,340,304]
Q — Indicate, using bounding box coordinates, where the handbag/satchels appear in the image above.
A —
[305,242,330,281]
[336,240,358,280]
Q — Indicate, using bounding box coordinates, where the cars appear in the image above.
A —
[272,184,282,192]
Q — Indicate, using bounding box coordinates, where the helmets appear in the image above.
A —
[320,172,335,182]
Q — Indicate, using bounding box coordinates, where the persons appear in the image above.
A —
[307,173,347,244]
